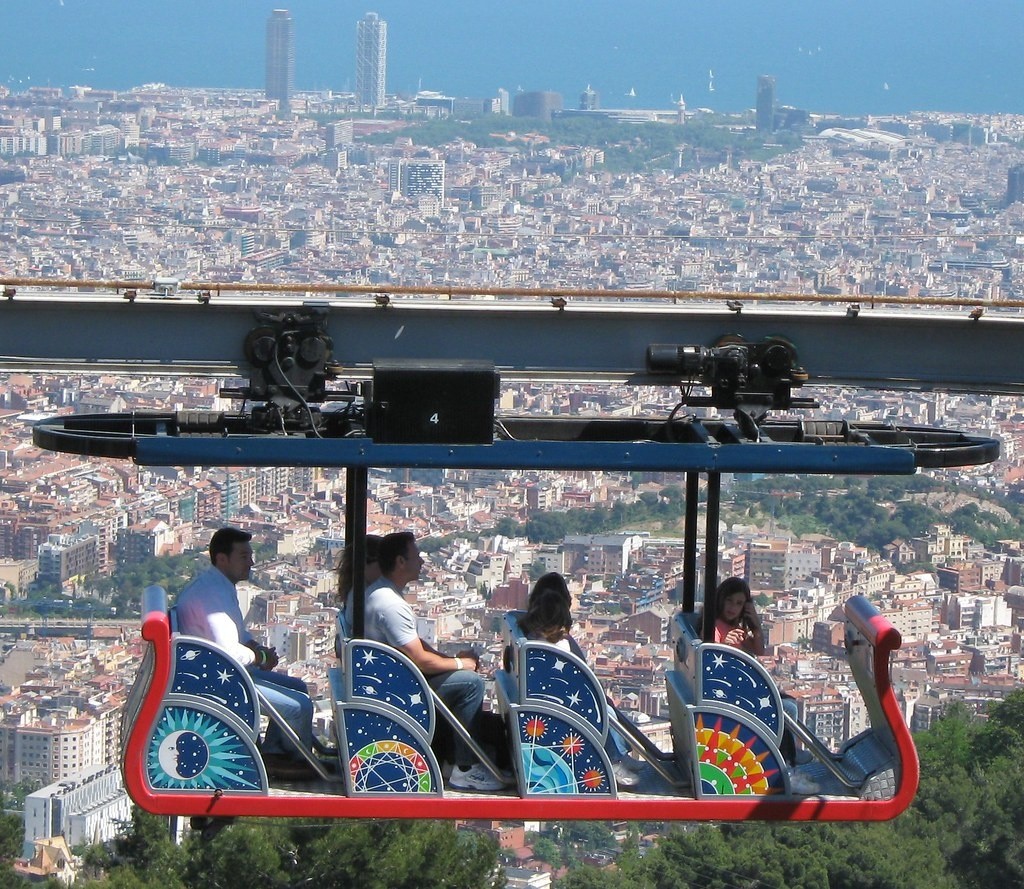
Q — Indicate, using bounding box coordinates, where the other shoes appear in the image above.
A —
[267,760,336,780]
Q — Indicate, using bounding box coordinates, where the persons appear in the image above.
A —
[517,591,645,786]
[529,572,572,610]
[698,577,821,794]
[338,534,384,640]
[780,692,813,765]
[364,532,515,791]
[176,527,335,781]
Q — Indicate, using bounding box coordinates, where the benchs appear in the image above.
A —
[494,610,618,800]
[665,612,793,800]
[328,609,444,798]
[171,606,271,744]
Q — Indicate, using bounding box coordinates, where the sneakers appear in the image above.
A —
[789,768,821,795]
[795,747,814,764]
[612,762,639,786]
[622,754,651,772]
[441,762,513,790]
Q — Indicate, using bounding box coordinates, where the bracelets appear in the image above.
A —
[260,650,267,665]
[455,658,464,670]
[254,645,262,651]
